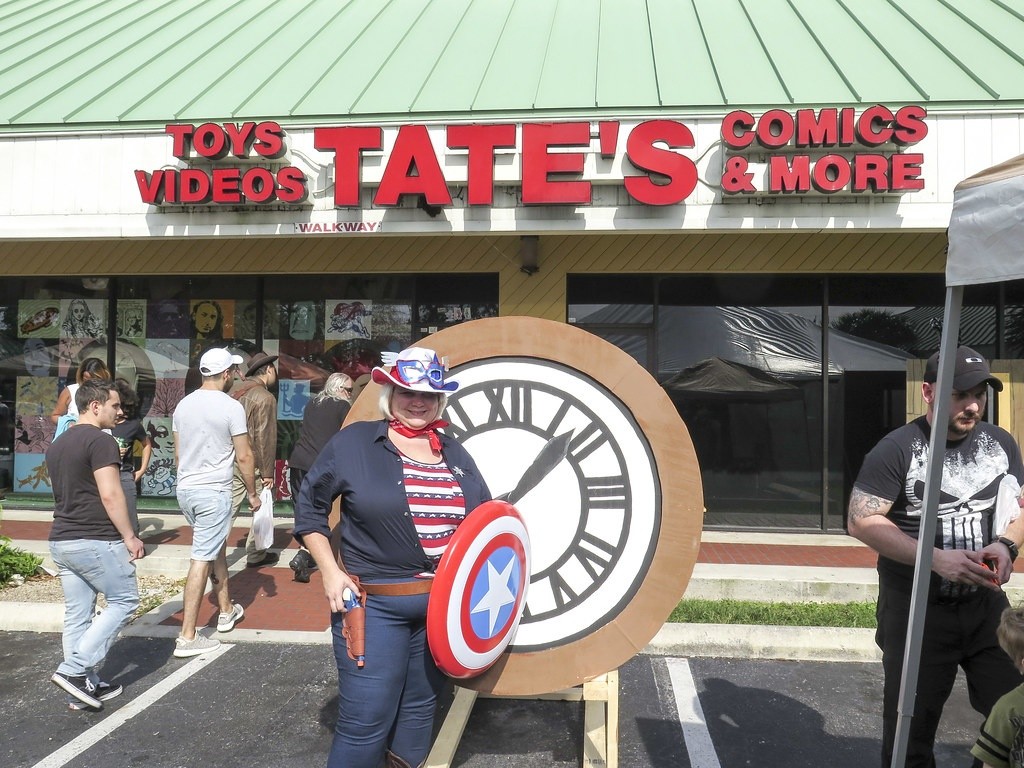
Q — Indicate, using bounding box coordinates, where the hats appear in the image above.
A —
[246,352,278,376]
[371,346,458,393]
[199,348,243,377]
[924,343,1003,391]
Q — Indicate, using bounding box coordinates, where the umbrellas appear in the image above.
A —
[0,339,190,373]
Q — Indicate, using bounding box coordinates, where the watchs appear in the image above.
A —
[997,537,1018,564]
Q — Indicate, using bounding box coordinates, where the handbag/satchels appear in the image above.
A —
[251,484,274,551]
[274,460,293,502]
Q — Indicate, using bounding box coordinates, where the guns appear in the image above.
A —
[342,586,366,668]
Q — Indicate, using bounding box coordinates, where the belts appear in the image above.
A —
[360,580,433,595]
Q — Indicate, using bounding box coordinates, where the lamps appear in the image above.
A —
[519,233,540,278]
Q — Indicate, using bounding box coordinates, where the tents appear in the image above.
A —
[890,155,1024,768]
[576,303,920,493]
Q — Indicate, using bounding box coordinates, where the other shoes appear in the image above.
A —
[247,552,279,567]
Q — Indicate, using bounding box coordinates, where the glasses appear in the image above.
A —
[344,387,353,392]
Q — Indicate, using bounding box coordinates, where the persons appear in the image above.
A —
[173,348,262,657]
[292,346,493,768]
[228,352,279,568]
[288,373,354,582]
[848,345,1024,768]
[112,384,151,539]
[51,357,111,428]
[970,605,1024,768]
[46,379,144,710]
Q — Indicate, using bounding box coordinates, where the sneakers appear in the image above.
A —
[69,682,122,710]
[217,604,245,632]
[290,551,310,583]
[51,672,102,708]
[173,632,219,658]
